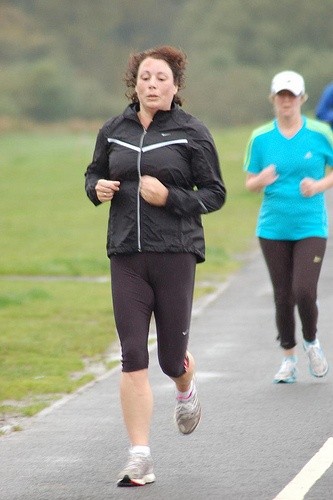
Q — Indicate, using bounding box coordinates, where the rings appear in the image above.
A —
[104,193,106,196]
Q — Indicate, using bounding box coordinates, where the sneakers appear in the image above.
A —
[273,356,298,383]
[175,374,201,434]
[117,453,154,487]
[302,338,329,377]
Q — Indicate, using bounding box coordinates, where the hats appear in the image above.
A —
[273,71,304,96]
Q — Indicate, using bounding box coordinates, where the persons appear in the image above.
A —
[85,49,226,487]
[243,70,333,383]
